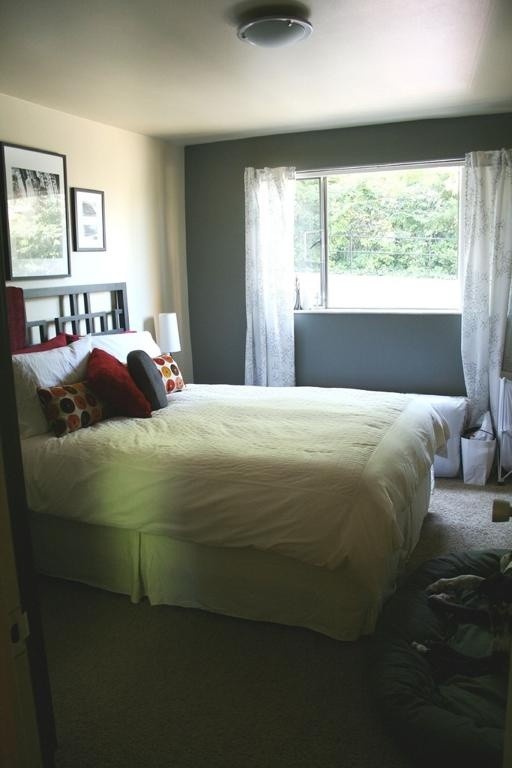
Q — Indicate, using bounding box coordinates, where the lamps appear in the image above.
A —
[236,4,314,49]
[152,312,183,356]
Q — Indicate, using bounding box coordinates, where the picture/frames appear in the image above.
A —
[0,141,72,281]
[70,187,107,252]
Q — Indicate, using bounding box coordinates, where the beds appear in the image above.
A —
[0,281,451,641]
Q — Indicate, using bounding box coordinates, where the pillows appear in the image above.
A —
[12,328,185,439]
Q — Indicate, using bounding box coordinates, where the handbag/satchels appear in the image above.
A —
[461,427,496,487]
